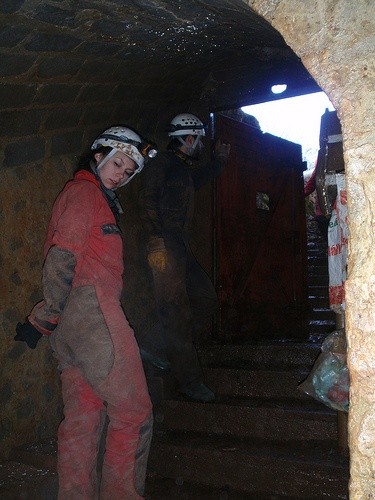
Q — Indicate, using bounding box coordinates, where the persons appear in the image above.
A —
[11,124,158,500]
[137,112,232,404]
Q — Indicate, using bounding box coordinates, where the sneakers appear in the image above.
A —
[174,376,215,403]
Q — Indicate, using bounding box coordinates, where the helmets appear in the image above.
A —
[163,113,206,139]
[91,125,149,172]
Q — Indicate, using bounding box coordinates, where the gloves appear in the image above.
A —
[14,320,41,351]
[146,237,168,273]
